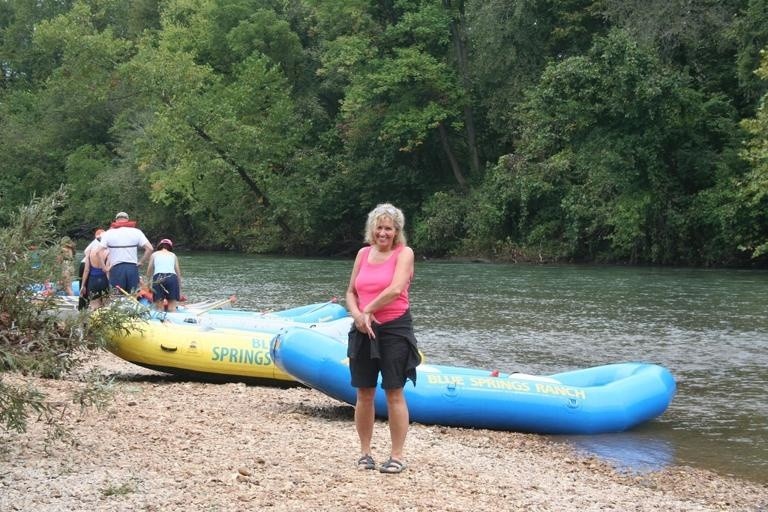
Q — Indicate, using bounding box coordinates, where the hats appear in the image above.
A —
[161,239,173,247]
[115,212,129,219]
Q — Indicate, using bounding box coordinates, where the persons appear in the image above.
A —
[53,237,76,295]
[77,229,106,311]
[147,238,181,313]
[96,212,154,297]
[346,201,424,475]
[79,237,111,308]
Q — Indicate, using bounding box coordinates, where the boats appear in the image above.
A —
[269,326,678,437]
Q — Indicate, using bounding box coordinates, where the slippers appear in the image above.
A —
[380,456,407,473]
[358,456,375,469]
